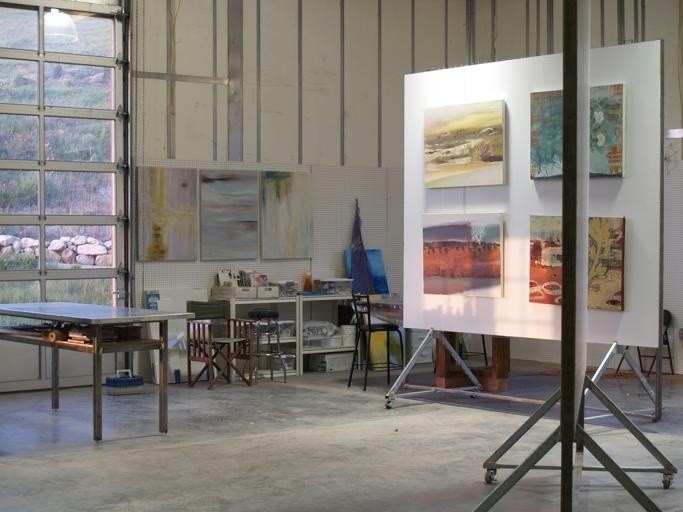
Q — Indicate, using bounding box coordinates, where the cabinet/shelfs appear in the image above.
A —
[208,295,362,376]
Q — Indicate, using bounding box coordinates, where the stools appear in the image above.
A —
[242,309,287,385]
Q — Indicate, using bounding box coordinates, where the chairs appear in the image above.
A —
[186,300,251,388]
[346,290,406,392]
[615,309,672,377]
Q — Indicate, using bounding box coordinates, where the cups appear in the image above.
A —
[320,281,336,294]
[304,271,312,292]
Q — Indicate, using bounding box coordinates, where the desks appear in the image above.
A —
[0,303,195,442]
